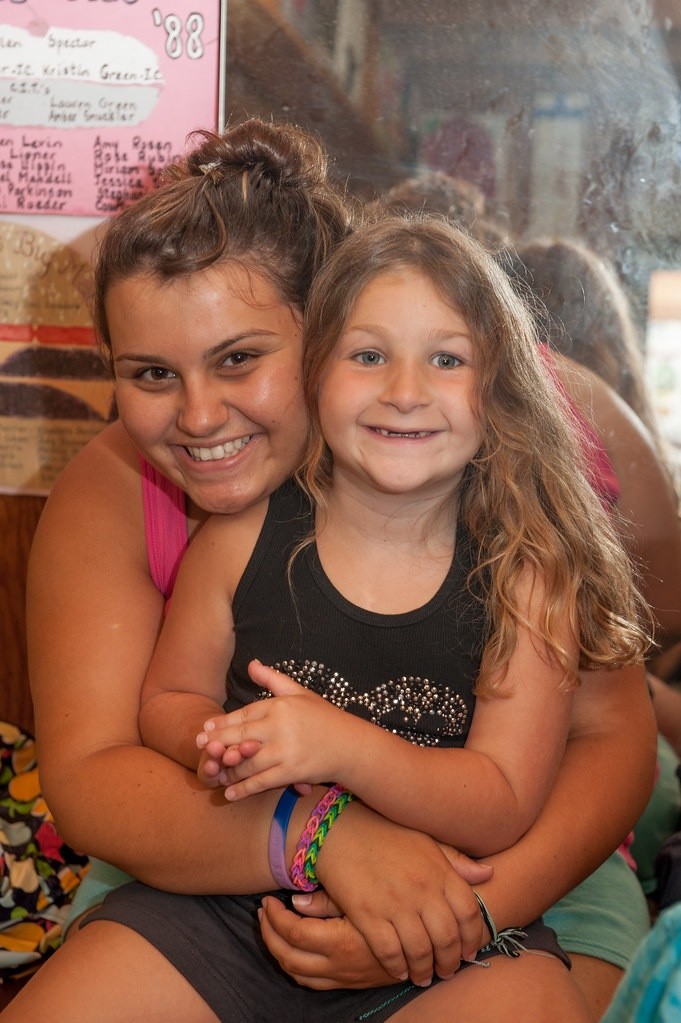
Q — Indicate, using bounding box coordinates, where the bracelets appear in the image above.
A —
[261,783,360,897]
[462,884,523,962]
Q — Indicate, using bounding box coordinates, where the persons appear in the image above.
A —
[1,207,608,1022]
[15,95,669,1020]
[369,166,680,897]
[511,229,663,457]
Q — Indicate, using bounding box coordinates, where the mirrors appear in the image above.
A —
[217,1,676,506]
